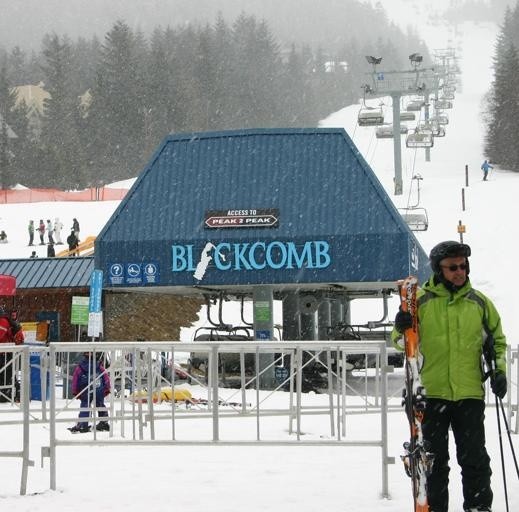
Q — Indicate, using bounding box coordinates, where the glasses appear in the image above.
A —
[439,263,468,271]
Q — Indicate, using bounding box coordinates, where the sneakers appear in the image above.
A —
[96,421,110,431]
[71,423,90,431]
[29,240,63,246]
[465,505,493,512]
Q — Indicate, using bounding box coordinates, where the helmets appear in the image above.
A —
[83,352,98,357]
[429,240,472,265]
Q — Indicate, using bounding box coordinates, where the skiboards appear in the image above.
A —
[395,276,429,512]
[134,398,251,409]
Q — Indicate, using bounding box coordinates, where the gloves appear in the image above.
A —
[103,388,110,397]
[11,324,21,335]
[492,373,507,398]
[395,306,413,333]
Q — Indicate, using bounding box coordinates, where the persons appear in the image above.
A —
[0,230,7,241]
[72,351,112,432]
[481,160,494,181]
[391,240,508,511]
[28,218,81,258]
[0,298,25,402]
